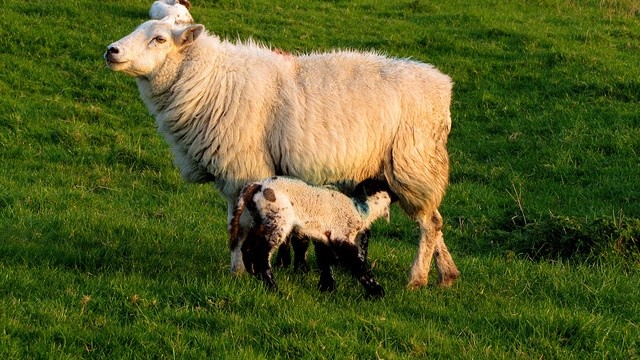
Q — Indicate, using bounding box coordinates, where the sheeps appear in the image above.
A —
[229,176,400,299]
[104,1,462,290]
